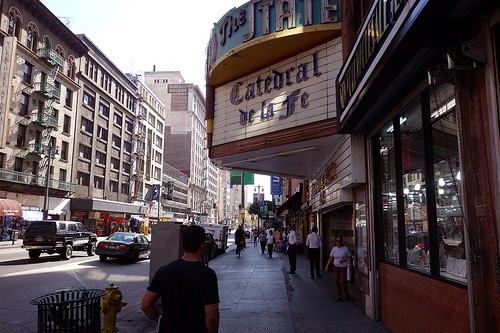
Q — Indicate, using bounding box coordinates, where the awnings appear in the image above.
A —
[276,192,300,217]
[134,217,146,223]
[0,198,23,216]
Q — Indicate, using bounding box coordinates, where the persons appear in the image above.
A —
[235,225,248,258]
[325,236,353,302]
[140,226,220,333]
[306,226,323,280]
[286,225,299,274]
[251,225,298,258]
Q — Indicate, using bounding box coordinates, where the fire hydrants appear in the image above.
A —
[99,282,128,333]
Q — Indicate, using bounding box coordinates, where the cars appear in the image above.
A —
[94,231,151,264]
[202,232,219,265]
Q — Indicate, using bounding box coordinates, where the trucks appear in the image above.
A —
[201,223,229,253]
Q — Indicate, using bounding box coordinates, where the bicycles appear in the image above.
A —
[235,237,244,257]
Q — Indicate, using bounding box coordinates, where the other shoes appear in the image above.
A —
[335,297,343,301]
[311,277,315,279]
[288,271,295,274]
[317,274,322,277]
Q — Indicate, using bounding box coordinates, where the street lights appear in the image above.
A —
[254,184,265,243]
[40,145,60,220]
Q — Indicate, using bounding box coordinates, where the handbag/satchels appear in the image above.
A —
[346,262,352,281]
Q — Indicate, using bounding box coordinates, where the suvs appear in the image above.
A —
[21,219,98,261]
